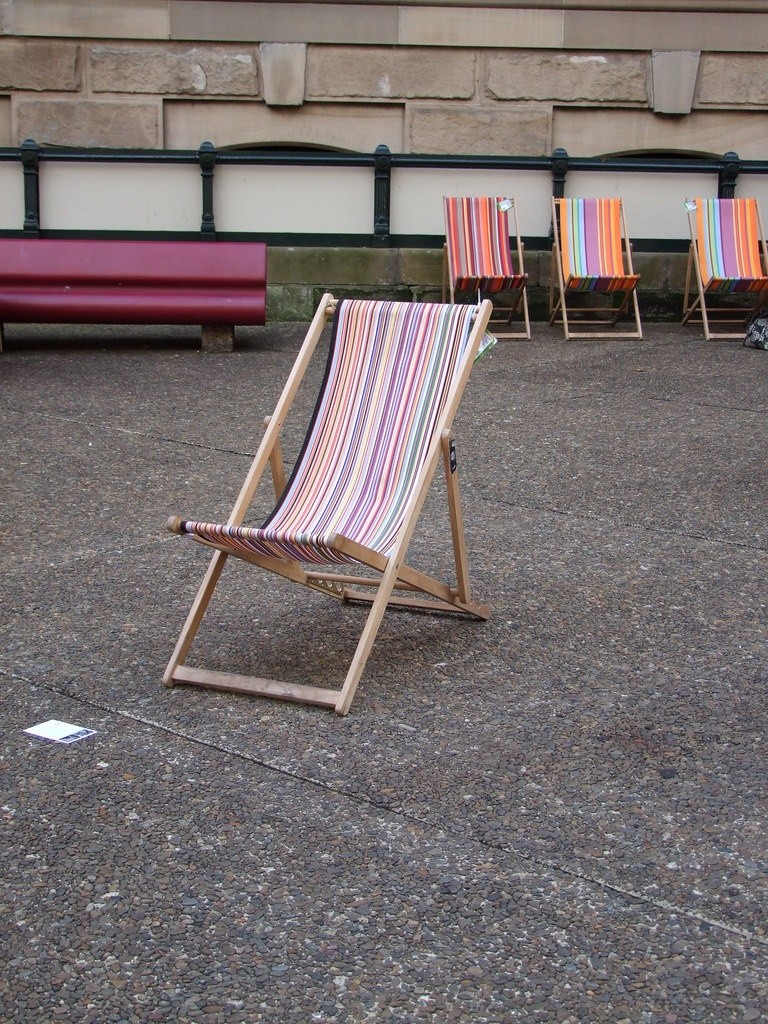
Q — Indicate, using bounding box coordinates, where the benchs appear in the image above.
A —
[0,239,268,354]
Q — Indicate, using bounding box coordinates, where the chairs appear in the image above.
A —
[163,292,494,716]
[682,197,768,340]
[443,196,530,339]
[549,195,643,341]
